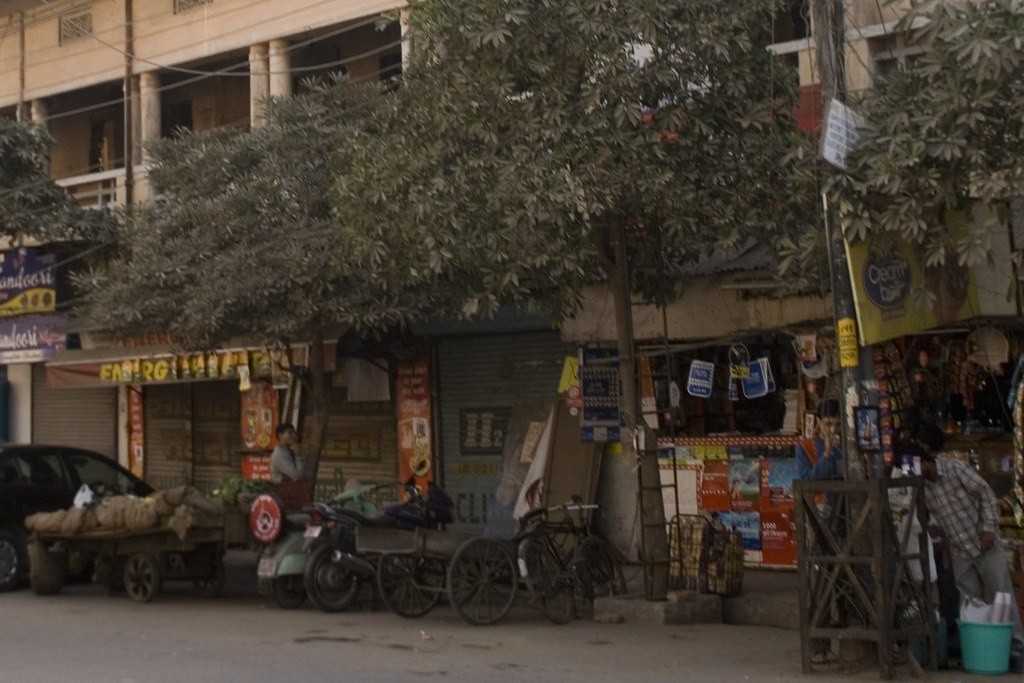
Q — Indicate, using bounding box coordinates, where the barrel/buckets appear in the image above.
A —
[955,619,1013,675]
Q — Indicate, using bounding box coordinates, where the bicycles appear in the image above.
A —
[515,493,627,623]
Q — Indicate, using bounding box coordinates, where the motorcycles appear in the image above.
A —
[299,477,460,616]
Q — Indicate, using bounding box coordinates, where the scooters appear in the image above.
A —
[246,477,397,609]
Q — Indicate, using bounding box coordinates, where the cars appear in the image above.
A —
[0,444,161,595]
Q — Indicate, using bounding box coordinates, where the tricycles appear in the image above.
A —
[354,487,616,623]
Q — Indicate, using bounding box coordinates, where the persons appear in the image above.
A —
[268,423,310,486]
[791,397,855,659]
[889,439,1024,670]
